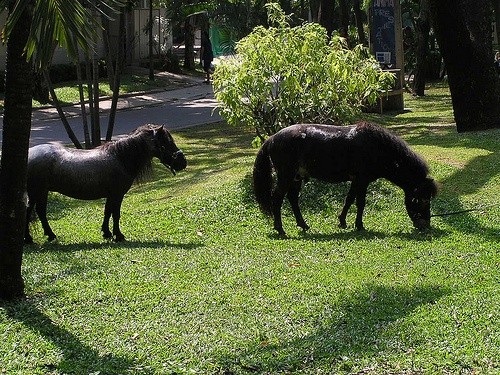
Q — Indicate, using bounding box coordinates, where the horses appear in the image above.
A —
[253,119,440,235]
[26,119,187,244]
